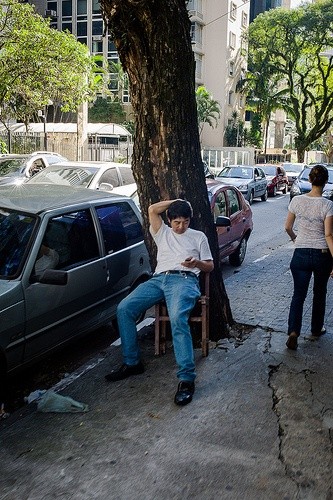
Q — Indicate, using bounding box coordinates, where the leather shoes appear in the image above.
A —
[105,361,144,381]
[174,381,195,406]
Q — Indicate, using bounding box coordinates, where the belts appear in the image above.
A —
[159,270,198,280]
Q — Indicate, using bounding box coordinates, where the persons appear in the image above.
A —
[33,243,59,275]
[105,199,215,406]
[284,165,333,350]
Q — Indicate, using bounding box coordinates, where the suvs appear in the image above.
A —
[0,151,68,186]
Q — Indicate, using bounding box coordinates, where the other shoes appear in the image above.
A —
[312,326,326,336]
[286,332,298,351]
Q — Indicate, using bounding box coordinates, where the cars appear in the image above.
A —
[290,165,333,201]
[0,185,153,379]
[130,179,253,265]
[254,164,288,196]
[216,165,268,204]
[20,161,137,197]
[280,163,307,187]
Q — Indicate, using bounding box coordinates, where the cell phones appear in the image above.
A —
[185,259,191,261]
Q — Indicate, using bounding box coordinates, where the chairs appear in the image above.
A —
[153,272,210,357]
